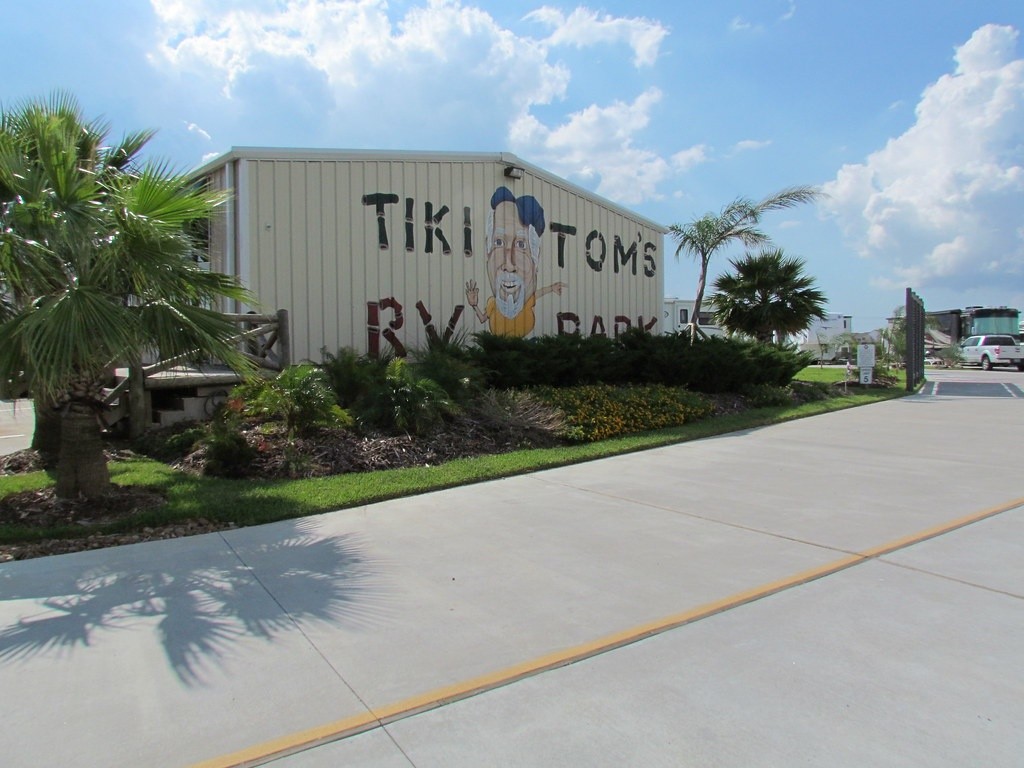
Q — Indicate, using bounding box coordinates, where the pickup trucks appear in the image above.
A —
[959,335,1024,371]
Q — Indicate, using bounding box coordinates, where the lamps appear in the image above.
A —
[503,166,525,181]
